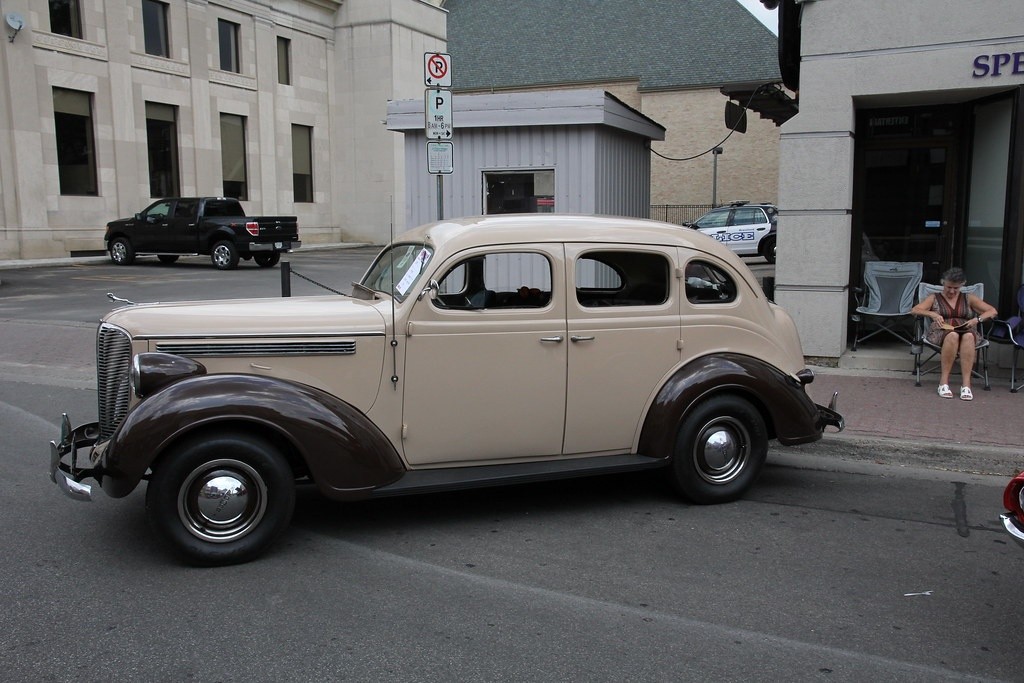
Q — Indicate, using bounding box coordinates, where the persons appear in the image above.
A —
[910,267,998,401]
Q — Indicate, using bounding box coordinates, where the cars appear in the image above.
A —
[49,212,845,568]
[678,199,779,262]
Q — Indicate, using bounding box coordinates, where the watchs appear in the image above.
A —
[976,316,983,323]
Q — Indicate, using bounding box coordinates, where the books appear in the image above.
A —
[938,322,971,333]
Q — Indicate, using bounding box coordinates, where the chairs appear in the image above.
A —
[851,261,923,355]
[910,283,989,389]
[993,285,1024,393]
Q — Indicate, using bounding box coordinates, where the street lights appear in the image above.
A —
[712,147,725,209]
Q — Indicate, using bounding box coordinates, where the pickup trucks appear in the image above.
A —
[104,197,303,270]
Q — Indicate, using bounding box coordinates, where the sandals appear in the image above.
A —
[938,384,953,398]
[960,386,973,400]
